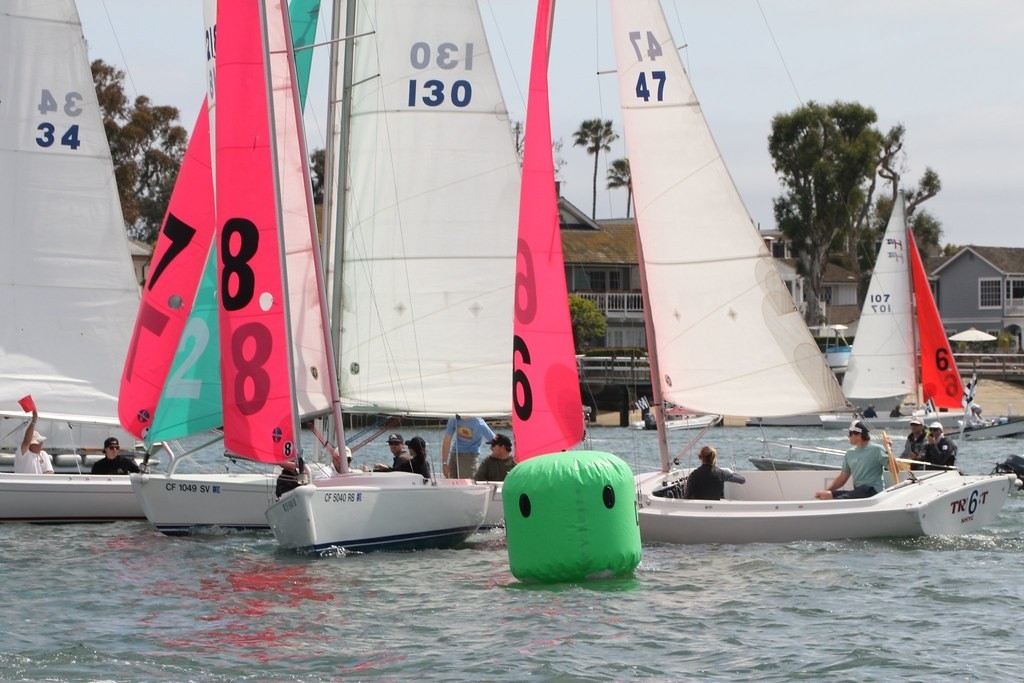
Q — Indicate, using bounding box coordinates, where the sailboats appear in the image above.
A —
[118,1,341,526]
[0,0,143,516]
[510,3,1022,539]
[817,190,977,429]
[265,0,527,552]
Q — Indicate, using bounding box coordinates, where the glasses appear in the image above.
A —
[911,423,920,427]
[389,442,401,446]
[849,430,859,436]
[348,457,351,463]
[108,446,121,450]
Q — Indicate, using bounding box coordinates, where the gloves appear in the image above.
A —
[721,467,734,474]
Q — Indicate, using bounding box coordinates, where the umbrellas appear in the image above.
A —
[948,327,998,342]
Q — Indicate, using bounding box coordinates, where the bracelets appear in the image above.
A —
[32,421,35,425]
[442,462,447,464]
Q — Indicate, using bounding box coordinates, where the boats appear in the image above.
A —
[1,350,159,465]
[1005,454,1024,476]
[943,415,1023,440]
[746,409,965,430]
[807,302,909,409]
[748,438,958,471]
[628,414,724,430]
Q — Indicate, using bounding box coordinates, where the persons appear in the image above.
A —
[901,417,957,470]
[815,417,899,499]
[890,405,904,417]
[405,436,431,478]
[360,432,412,472]
[276,461,301,497]
[442,415,497,478]
[92,438,140,475]
[864,404,877,418]
[474,434,517,481]
[683,446,745,500]
[14,411,54,474]
[316,445,355,477]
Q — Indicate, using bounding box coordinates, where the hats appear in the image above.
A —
[334,445,352,458]
[971,403,980,409]
[103,438,119,454]
[28,431,46,445]
[406,437,425,449]
[910,417,925,427]
[485,435,512,448]
[842,420,870,434]
[929,421,943,431]
[386,433,403,443]
[975,407,984,412]
